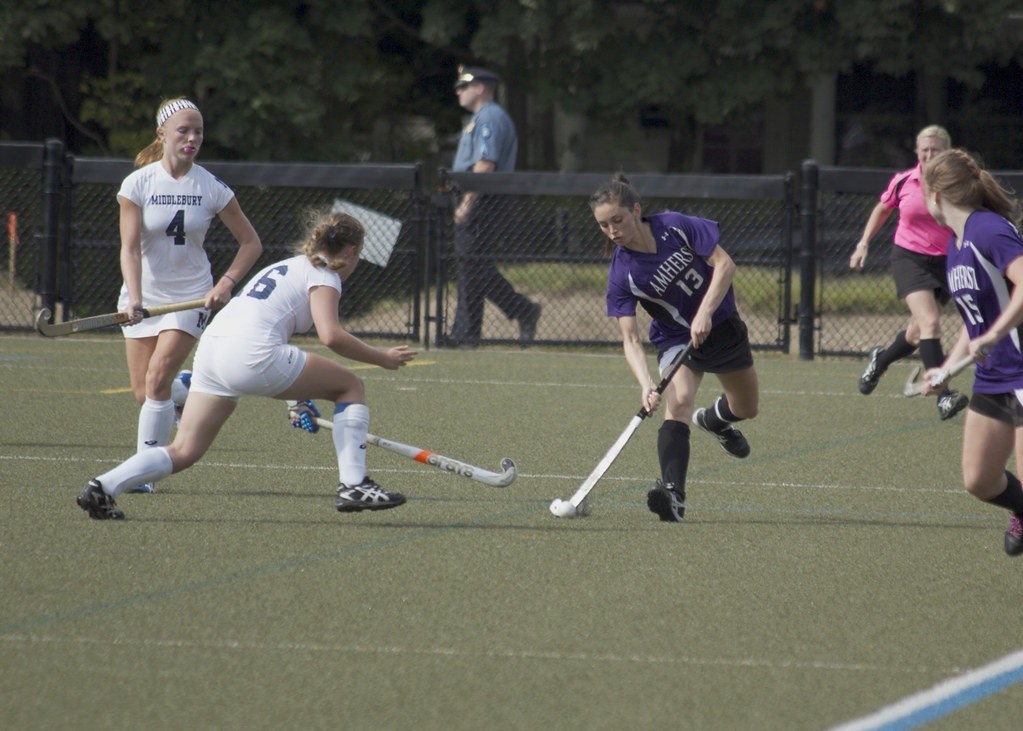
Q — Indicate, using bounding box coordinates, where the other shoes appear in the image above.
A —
[438,333,477,350]
[518,302,541,349]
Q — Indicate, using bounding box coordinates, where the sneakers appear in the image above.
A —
[858,346,889,395]
[1004,511,1023,555]
[173,370,193,429]
[693,407,750,459]
[336,472,407,513]
[646,478,685,522]
[78,478,125,520]
[937,388,969,420]
[127,480,156,493]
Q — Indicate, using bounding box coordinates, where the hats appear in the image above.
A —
[453,64,500,88]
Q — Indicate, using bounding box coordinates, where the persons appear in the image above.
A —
[74,212,419,519]
[591,173,760,521]
[433,57,542,346]
[115,97,264,492]
[919,149,1023,558]
[848,125,969,420]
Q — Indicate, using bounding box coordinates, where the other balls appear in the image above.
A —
[557,501,576,518]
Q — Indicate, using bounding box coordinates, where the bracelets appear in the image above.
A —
[224,275,236,284]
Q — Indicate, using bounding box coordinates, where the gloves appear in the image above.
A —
[286,397,321,433]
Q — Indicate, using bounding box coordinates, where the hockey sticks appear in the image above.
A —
[288,409,519,489]
[549,339,694,517]
[904,347,990,398]
[35,298,207,338]
